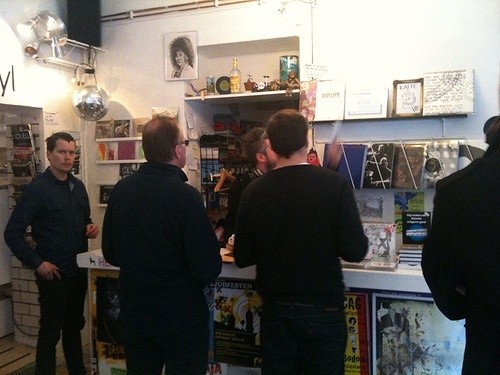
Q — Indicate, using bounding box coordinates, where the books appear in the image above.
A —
[324,139,462,270]
[10,122,81,241]
[94,106,180,204]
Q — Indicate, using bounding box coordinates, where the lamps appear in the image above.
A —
[16,10,68,46]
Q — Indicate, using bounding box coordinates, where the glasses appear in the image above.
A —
[179,140,189,146]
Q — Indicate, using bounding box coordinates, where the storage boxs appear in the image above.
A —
[201,146,248,185]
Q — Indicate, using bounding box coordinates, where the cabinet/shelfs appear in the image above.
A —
[95,137,147,208]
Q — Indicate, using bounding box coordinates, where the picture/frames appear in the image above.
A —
[391,78,424,117]
[164,31,199,81]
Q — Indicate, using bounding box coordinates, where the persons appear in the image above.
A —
[233,108,368,375]
[421,116,499,375]
[223,128,277,254]
[3,132,99,375]
[101,114,223,375]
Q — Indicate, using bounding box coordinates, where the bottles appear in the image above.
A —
[264,76,270,91]
[230,58,241,93]
[279,56,300,89]
[206,76,215,95]
